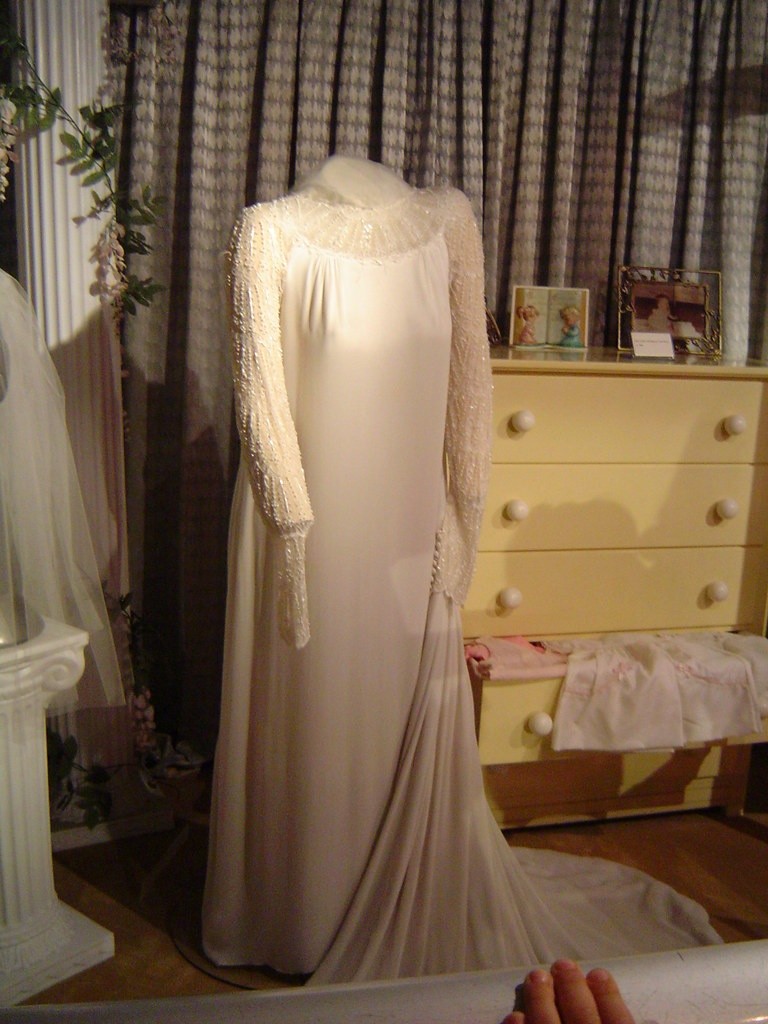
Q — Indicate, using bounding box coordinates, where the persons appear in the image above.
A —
[197,158,490,973]
[501,958,635,1024]
[648,294,678,334]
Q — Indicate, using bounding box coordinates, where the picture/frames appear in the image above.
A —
[618,265,722,356]
[509,285,589,353]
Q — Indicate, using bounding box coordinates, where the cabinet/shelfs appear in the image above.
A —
[462,344,768,830]
